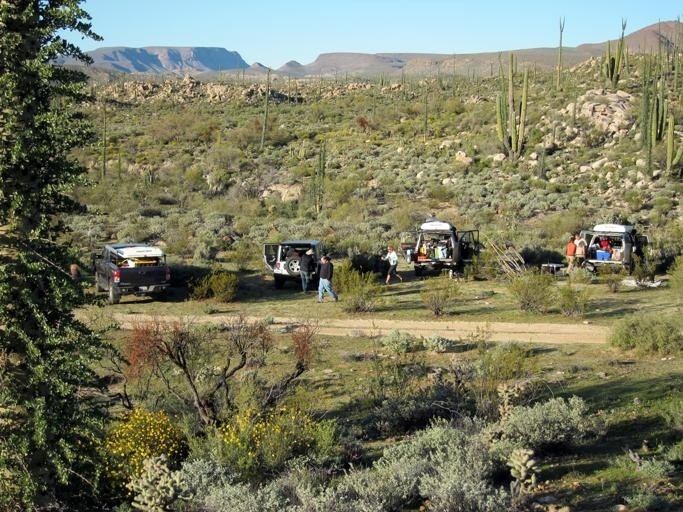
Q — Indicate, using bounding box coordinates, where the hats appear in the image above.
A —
[305,248,316,255]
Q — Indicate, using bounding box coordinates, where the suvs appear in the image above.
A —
[89,239,173,306]
[575,221,650,276]
[393,219,481,275]
[261,238,330,288]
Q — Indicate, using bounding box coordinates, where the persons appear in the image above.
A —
[574,232,588,266]
[318,256,338,302]
[565,237,576,276]
[301,250,313,292]
[381,246,402,284]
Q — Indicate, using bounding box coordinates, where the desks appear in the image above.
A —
[538,262,564,280]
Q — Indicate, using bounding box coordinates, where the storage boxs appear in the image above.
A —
[595,249,605,259]
[612,248,623,261]
[434,247,448,259]
[604,253,612,261]
[134,259,156,267]
